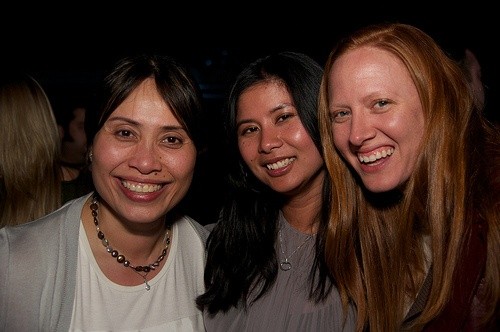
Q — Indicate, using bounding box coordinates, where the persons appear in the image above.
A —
[0,56,210,331]
[318,24,500,332]
[435,38,484,109]
[196,53,357,332]
[0,74,63,228]
[52,97,95,205]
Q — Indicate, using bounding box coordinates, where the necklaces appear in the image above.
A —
[90,192,169,284]
[277,221,318,271]
